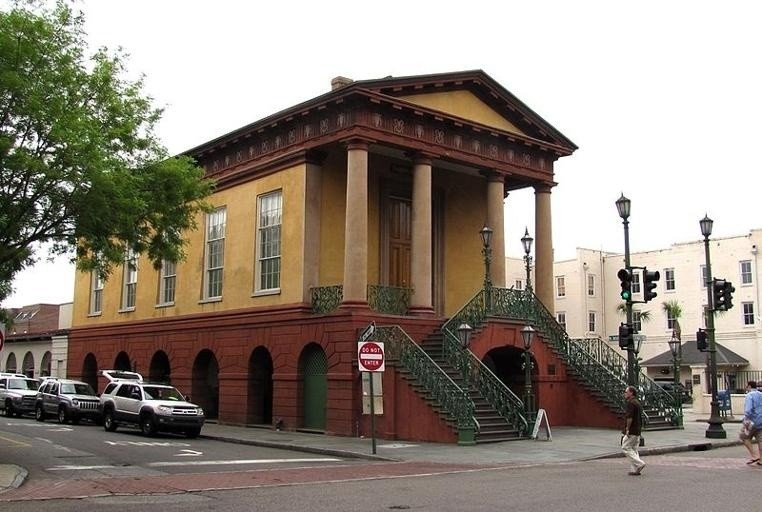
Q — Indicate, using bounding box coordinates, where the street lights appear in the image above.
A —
[458,309,475,445]
[633,324,645,391]
[520,226,533,316]
[668,332,684,429]
[477,220,494,312]
[616,191,645,448]
[697,212,726,439]
[518,320,537,438]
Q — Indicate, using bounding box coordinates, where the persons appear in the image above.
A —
[620,385,646,476]
[738,381,762,466]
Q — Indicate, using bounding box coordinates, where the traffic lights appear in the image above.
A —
[696,330,708,349]
[619,327,634,348]
[645,270,663,301]
[713,280,726,308]
[726,281,736,307]
[617,268,632,301]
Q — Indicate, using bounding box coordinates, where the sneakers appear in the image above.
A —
[636,463,646,472]
[746,457,762,466]
[629,472,642,476]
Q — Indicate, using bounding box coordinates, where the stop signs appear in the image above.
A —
[357,340,385,372]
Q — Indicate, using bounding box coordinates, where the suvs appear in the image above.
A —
[35,375,101,424]
[97,369,204,438]
[1,373,41,417]
[647,380,692,407]
